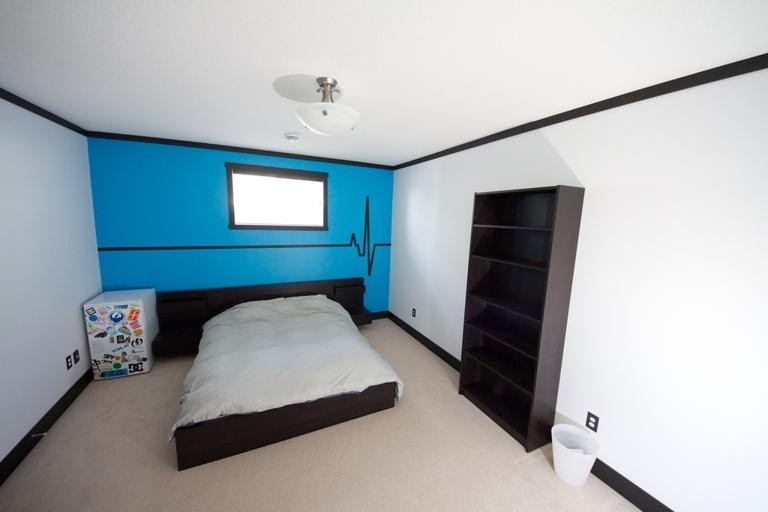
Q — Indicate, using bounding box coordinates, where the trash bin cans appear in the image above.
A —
[549,422,603,486]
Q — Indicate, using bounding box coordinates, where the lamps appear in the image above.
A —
[293,76,360,136]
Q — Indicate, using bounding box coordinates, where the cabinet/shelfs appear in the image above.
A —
[460,184,584,452]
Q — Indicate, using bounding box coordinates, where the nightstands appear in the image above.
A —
[84,288,161,380]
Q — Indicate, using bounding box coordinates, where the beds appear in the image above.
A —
[167,294,404,470]
